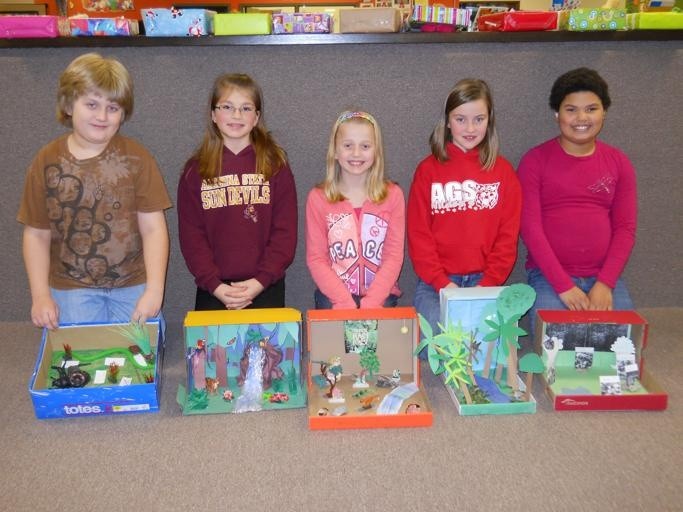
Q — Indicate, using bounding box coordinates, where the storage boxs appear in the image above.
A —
[438,287,539,413]
[305,307,435,427]
[181,307,305,412]
[29,321,164,420]
[535,308,668,411]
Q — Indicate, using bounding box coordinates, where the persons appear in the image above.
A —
[515,68,634,353]
[176,73,299,312]
[303,109,406,310]
[15,52,174,342]
[406,76,524,356]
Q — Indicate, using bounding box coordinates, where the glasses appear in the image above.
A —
[215,103,256,114]
